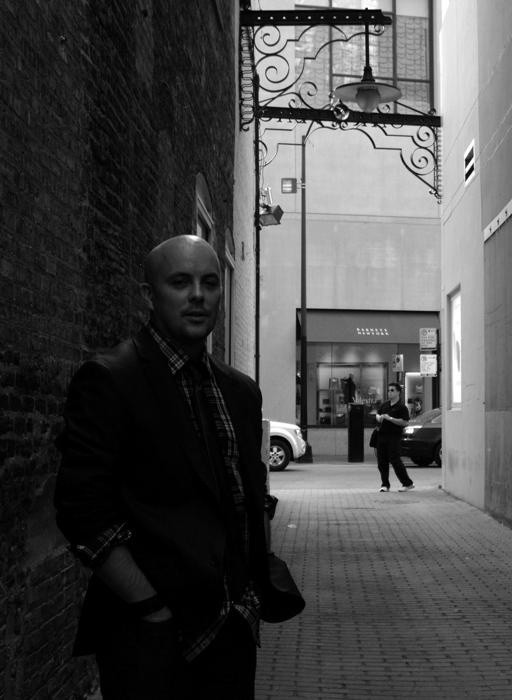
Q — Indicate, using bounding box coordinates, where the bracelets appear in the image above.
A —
[126,592,166,615]
[389,415,394,422]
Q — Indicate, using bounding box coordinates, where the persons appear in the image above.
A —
[374,384,416,493]
[343,373,356,413]
[52,235,308,697]
[411,397,423,415]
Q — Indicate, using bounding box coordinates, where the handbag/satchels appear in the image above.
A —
[369,427,379,448]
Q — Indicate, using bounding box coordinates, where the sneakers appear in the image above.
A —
[380,486,389,492]
[398,484,415,492]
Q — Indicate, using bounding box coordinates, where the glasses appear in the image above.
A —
[387,389,394,392]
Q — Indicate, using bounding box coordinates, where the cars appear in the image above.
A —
[269,417,307,472]
[400,406,442,468]
